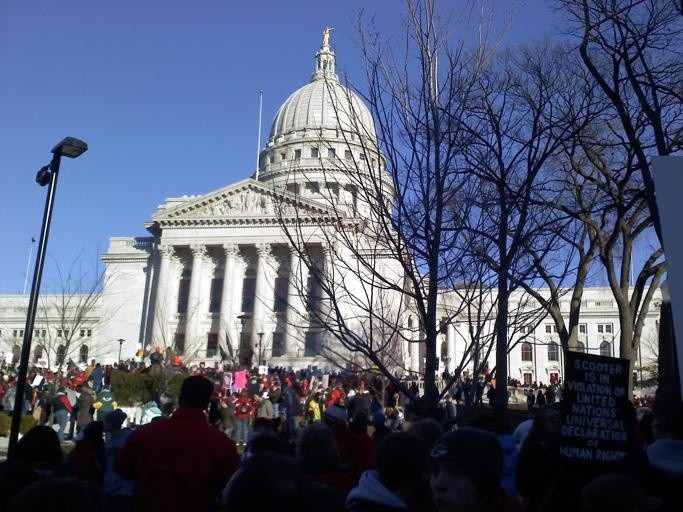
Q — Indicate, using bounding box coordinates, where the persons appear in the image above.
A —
[1,351,683,511]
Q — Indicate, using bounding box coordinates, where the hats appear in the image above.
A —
[324,404,346,423]
[105,409,127,428]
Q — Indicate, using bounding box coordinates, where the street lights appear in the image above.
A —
[6,135,90,472]
